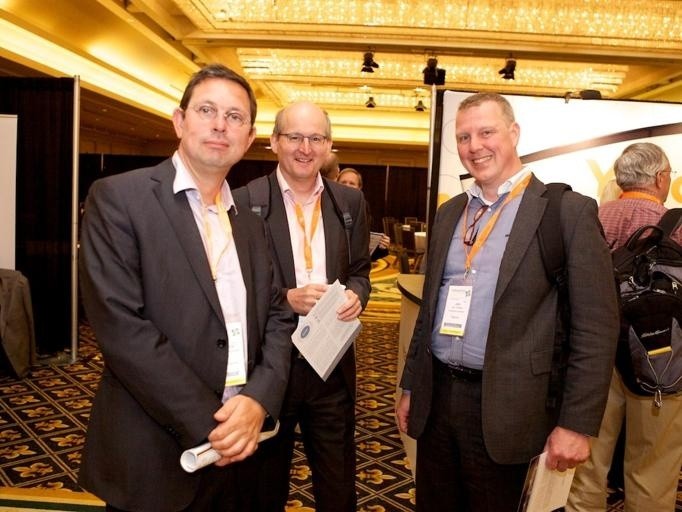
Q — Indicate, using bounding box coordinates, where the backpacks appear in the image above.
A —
[611,208,682,396]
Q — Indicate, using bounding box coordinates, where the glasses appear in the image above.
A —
[279,131,327,146]
[464,205,489,246]
[193,103,248,129]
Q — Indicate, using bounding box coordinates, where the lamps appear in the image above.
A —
[359,42,517,85]
[362,88,427,113]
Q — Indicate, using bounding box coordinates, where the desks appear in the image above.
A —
[0,266,38,378]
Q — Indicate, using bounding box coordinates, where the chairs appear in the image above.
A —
[376,210,427,276]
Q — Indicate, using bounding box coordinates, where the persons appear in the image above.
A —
[232,103,371,512]
[75,65,297,512]
[318,153,340,181]
[337,168,390,255]
[396,94,620,512]
[564,142,682,512]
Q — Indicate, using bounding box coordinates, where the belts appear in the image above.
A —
[432,356,481,382]
